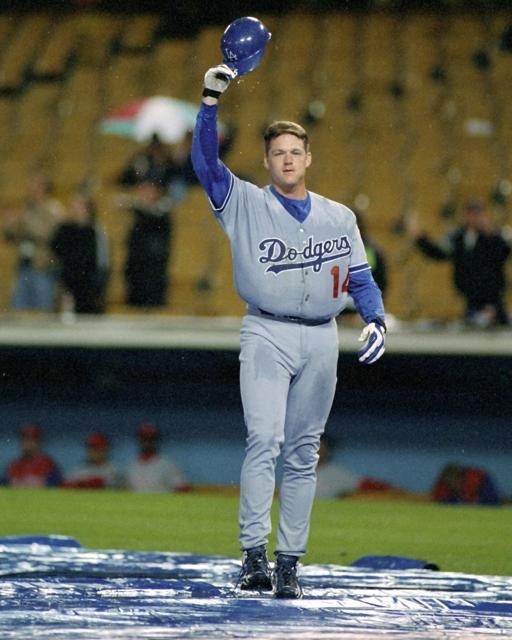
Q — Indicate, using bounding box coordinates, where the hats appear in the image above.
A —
[19,422,160,450]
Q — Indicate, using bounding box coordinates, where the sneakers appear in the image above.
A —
[239,545,272,590]
[272,554,301,597]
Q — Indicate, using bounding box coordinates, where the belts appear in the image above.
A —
[260,308,329,325]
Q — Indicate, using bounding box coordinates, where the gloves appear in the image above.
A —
[201,63,236,100]
[356,318,388,366]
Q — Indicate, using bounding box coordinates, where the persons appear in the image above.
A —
[2,425,60,490]
[52,192,111,312]
[63,432,119,488]
[414,194,510,323]
[1,178,62,311]
[169,124,238,189]
[311,432,360,500]
[126,422,188,490]
[118,141,175,185]
[124,182,177,305]
[192,65,389,596]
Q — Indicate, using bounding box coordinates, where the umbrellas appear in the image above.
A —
[91,93,223,149]
